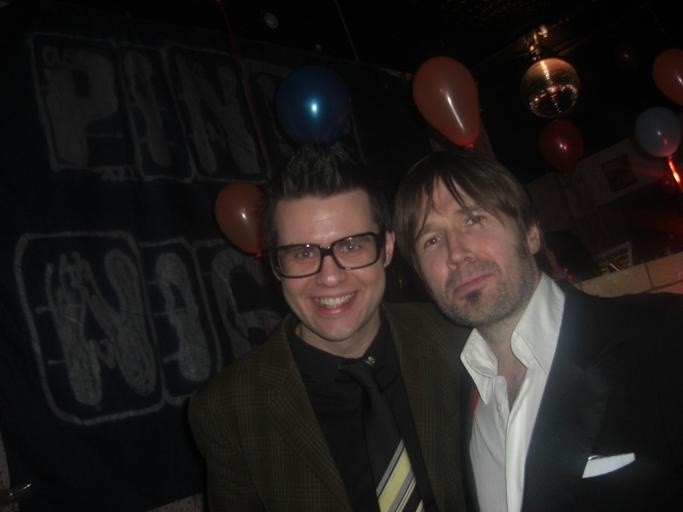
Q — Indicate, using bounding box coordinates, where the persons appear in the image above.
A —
[188,142,481,512]
[390,151,683,512]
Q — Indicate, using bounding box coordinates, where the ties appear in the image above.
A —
[339,351,425,512]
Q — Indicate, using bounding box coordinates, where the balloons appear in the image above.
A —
[213,179,271,259]
[636,105,681,158]
[413,55,481,149]
[652,47,682,106]
[538,118,586,178]
[616,45,638,65]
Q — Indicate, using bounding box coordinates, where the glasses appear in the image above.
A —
[269,231,386,278]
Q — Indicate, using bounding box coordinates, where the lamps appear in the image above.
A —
[513,24,582,120]
